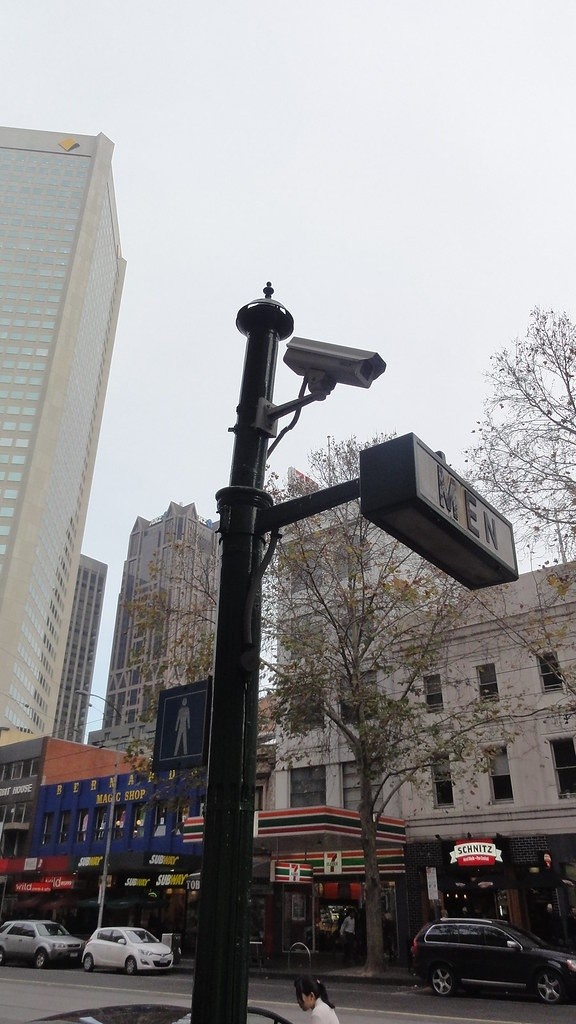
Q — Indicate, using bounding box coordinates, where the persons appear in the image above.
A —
[383,914,399,964]
[295,975,339,1024]
[339,909,356,968]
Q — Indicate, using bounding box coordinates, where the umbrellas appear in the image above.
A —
[16,889,161,911]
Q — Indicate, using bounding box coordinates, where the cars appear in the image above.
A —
[0,920,86,969]
[82,926,174,976]
[21,1004,293,1024]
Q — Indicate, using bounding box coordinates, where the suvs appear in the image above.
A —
[407,918,576,1005]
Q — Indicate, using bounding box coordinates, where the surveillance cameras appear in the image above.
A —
[285,336,387,390]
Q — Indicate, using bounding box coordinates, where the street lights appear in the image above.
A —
[74,689,123,929]
